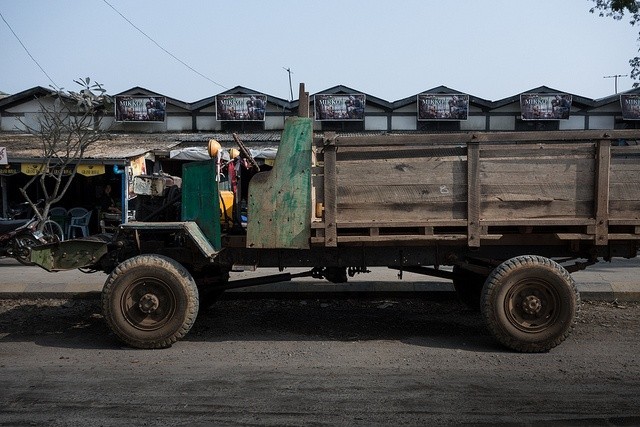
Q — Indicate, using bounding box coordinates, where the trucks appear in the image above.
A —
[28,82,639,353]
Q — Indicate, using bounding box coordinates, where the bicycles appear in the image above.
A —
[0,198,64,243]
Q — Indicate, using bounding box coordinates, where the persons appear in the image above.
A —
[96,184,119,225]
[145,97,165,121]
[246,96,264,119]
[625,95,638,117]
[344,96,363,119]
[552,96,571,117]
[448,96,467,119]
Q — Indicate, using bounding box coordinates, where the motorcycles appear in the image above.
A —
[0,218,47,265]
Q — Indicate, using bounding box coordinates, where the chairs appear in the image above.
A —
[48,206,68,236]
[66,207,90,239]
[68,210,93,239]
